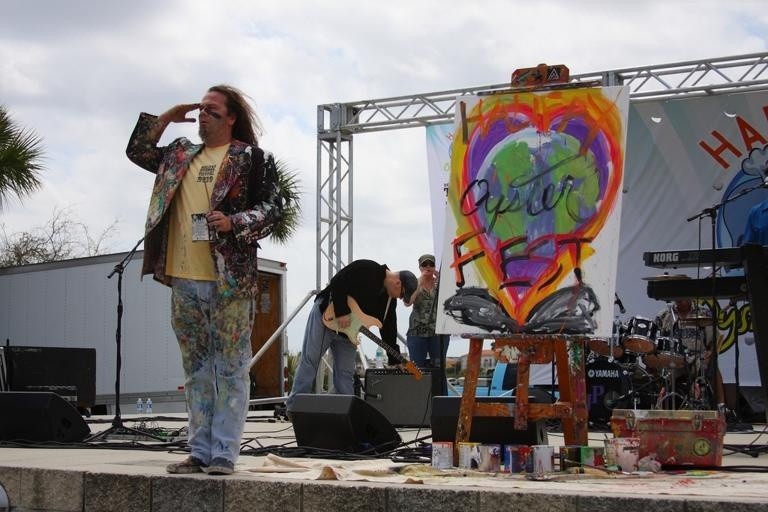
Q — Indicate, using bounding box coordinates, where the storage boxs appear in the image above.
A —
[610,408,728,470]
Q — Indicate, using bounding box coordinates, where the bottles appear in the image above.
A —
[375,344,387,368]
[135,397,152,416]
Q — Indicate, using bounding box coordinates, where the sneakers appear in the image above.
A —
[167,455,207,473]
[207,457,234,475]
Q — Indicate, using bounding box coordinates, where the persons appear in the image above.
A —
[284,259,417,422]
[404,255,451,369]
[743,200,768,246]
[653,274,730,412]
[125,84,284,476]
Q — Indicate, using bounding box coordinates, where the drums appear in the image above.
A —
[582,360,649,425]
[588,320,624,357]
[641,337,688,368]
[624,317,659,353]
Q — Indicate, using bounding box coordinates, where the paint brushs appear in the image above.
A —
[564,458,640,475]
[205,182,219,240]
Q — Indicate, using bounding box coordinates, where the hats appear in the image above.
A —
[400,271,418,304]
[418,254,435,268]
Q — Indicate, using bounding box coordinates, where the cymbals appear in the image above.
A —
[683,316,713,321]
[642,274,691,280]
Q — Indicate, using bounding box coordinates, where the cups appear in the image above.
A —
[430,441,454,468]
[603,438,642,473]
[503,445,557,476]
[459,440,502,473]
[561,445,603,476]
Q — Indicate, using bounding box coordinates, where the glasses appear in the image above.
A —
[421,261,435,267]
[400,283,404,299]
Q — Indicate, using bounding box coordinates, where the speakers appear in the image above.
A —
[0,391,91,443]
[288,393,403,456]
[0,345,97,416]
[364,367,448,428]
[430,396,549,445]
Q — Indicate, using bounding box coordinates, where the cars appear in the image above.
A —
[369,361,376,368]
[446,377,458,385]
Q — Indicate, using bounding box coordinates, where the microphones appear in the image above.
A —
[365,392,382,401]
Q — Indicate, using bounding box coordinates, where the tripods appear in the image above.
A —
[81,237,166,444]
[684,180,768,458]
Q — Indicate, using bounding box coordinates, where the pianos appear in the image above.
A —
[647,276,751,301]
[643,246,768,268]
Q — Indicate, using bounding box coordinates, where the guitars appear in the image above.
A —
[322,295,422,382]
